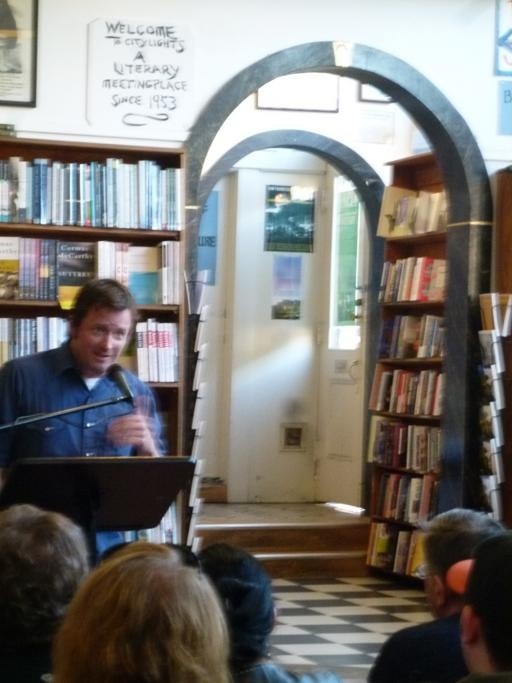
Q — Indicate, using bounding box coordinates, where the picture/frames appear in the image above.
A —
[255,55,396,112]
[0,0,39,106]
[495,0,512,74]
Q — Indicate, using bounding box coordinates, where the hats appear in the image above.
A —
[448,532,512,604]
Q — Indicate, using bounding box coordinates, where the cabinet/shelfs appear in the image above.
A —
[0,133,190,560]
[362,153,497,583]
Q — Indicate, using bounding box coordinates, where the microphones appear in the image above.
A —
[106,363,137,410]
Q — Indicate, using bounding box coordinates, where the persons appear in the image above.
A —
[47,539,239,682]
[438,530,511,681]
[1,501,97,682]
[187,540,349,682]
[360,504,511,682]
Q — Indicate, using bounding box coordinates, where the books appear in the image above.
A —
[475,292,511,522]
[363,184,450,586]
[0,316,179,382]
[183,269,210,556]
[1,236,181,305]
[0,156,183,231]
[125,498,178,544]
